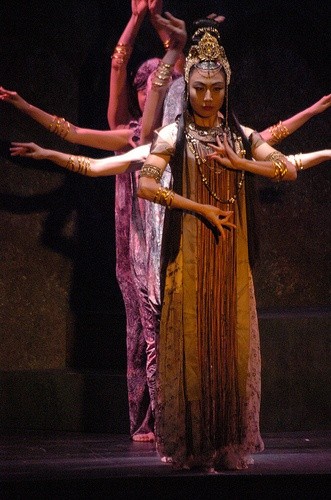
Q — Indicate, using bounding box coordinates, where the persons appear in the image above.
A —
[135,19,298,472]
[0,0,331,463]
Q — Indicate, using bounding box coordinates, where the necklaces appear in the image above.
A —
[174,116,247,204]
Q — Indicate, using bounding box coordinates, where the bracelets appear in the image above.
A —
[48,115,71,139]
[152,185,174,206]
[285,153,304,171]
[112,43,132,65]
[163,37,171,51]
[269,121,290,143]
[270,159,289,183]
[152,60,175,87]
[64,155,92,177]
